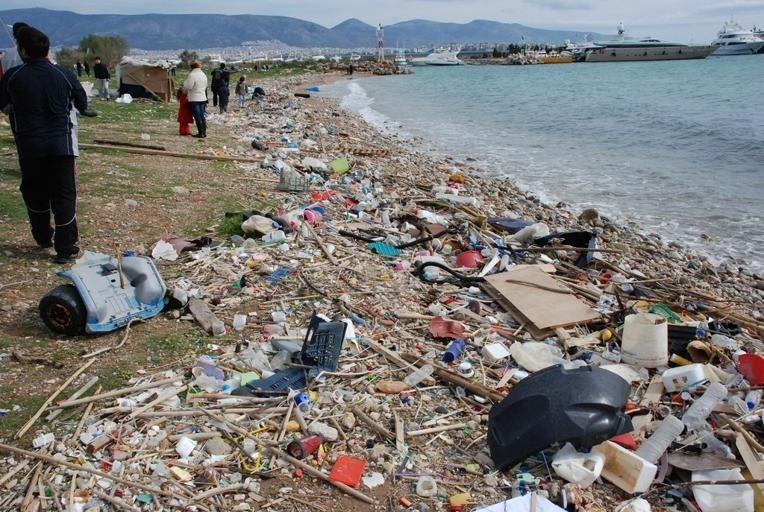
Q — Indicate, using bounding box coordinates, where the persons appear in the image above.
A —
[218,79,230,114]
[0,26,88,263]
[235,76,248,108]
[211,63,230,106]
[184,61,208,138]
[93,56,110,101]
[83,60,90,77]
[1,22,58,74]
[76,59,83,77]
[176,87,194,136]
[164,61,171,76]
[170,62,176,76]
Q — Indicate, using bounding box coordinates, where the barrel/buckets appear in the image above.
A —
[456,251,482,269]
[618,313,666,368]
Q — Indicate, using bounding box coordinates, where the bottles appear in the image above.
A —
[403,364,434,388]
[634,383,762,465]
[261,231,284,242]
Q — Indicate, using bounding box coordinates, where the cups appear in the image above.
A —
[284,433,323,460]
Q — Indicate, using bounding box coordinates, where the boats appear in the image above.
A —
[569,38,720,63]
[527,52,574,65]
[410,49,465,66]
[708,18,764,57]
[750,24,764,55]
[393,56,406,66]
